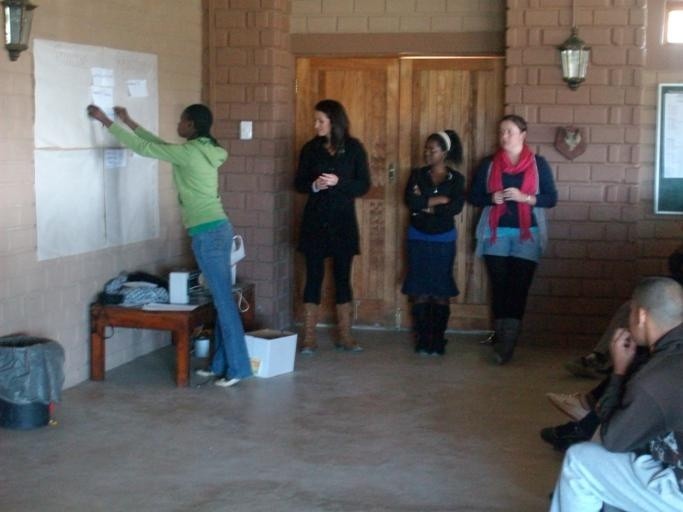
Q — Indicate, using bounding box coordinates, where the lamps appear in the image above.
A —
[0,0,38,60]
[558,25,592,89]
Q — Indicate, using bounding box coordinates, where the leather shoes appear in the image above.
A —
[215,378,240,386]
[196,368,216,376]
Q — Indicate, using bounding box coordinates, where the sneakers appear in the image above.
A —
[540,423,590,449]
[546,392,591,422]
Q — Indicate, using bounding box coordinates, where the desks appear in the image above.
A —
[91,285,256,385]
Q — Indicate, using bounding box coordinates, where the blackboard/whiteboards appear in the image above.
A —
[654,82,683,216]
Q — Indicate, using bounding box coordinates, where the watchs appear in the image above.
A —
[526,193,532,204]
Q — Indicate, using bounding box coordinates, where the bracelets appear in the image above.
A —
[311,181,319,193]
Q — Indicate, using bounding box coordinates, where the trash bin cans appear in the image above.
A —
[0,334,66,431]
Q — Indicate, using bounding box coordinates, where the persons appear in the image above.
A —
[547,276,683,511]
[88,103,255,386]
[540,396,606,455]
[402,130,467,355]
[469,114,559,365]
[294,99,373,353]
[563,245,683,378]
[548,338,651,421]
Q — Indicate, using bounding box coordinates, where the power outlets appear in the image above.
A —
[240,121,252,139]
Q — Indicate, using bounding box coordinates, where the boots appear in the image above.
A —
[300,304,319,354]
[431,304,451,357]
[412,303,432,357]
[334,302,365,353]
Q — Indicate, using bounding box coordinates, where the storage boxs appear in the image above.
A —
[244,328,298,376]
